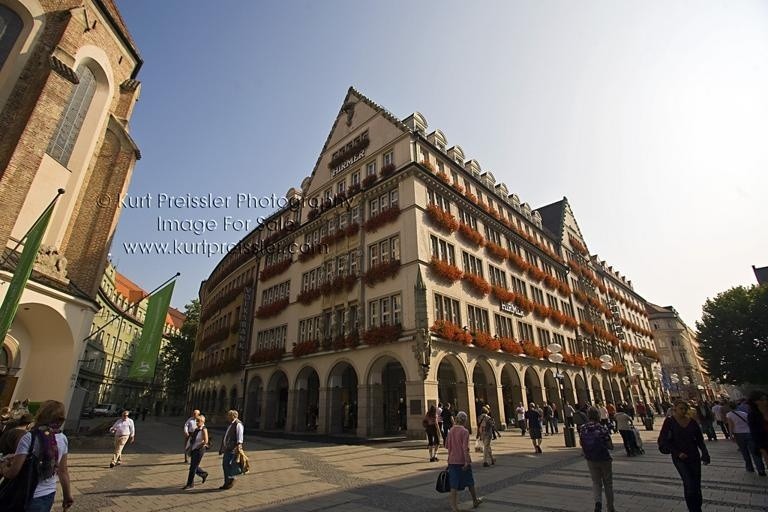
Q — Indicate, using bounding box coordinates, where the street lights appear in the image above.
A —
[650,365,692,416]
[549,336,574,449]
[631,362,654,421]
[600,353,620,418]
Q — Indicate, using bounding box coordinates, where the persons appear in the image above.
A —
[0,399,74,512]
[181,415,210,491]
[109,410,136,468]
[219,410,244,490]
[184,410,201,463]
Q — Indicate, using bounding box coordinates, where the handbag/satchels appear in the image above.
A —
[0,430,42,512]
[434,466,454,492]
[422,419,430,428]
[227,450,250,477]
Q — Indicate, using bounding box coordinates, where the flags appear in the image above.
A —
[127,281,174,382]
[0,202,57,342]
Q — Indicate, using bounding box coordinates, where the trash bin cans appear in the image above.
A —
[563,427,576,447]
[646,418,653,430]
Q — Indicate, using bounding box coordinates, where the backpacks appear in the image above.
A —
[657,434,674,454]
[580,423,603,462]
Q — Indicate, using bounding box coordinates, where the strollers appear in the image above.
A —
[621,424,646,457]
[603,415,619,435]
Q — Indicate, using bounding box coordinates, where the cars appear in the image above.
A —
[79,403,138,423]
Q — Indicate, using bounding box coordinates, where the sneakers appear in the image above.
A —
[220,482,233,491]
[536,444,543,454]
[110,461,121,469]
[472,496,488,509]
[482,461,490,467]
[492,457,497,466]
[593,502,603,512]
[429,456,440,462]
[609,416,654,435]
[746,467,767,476]
[182,482,195,490]
[520,428,558,436]
[708,435,731,441]
[202,472,208,484]
